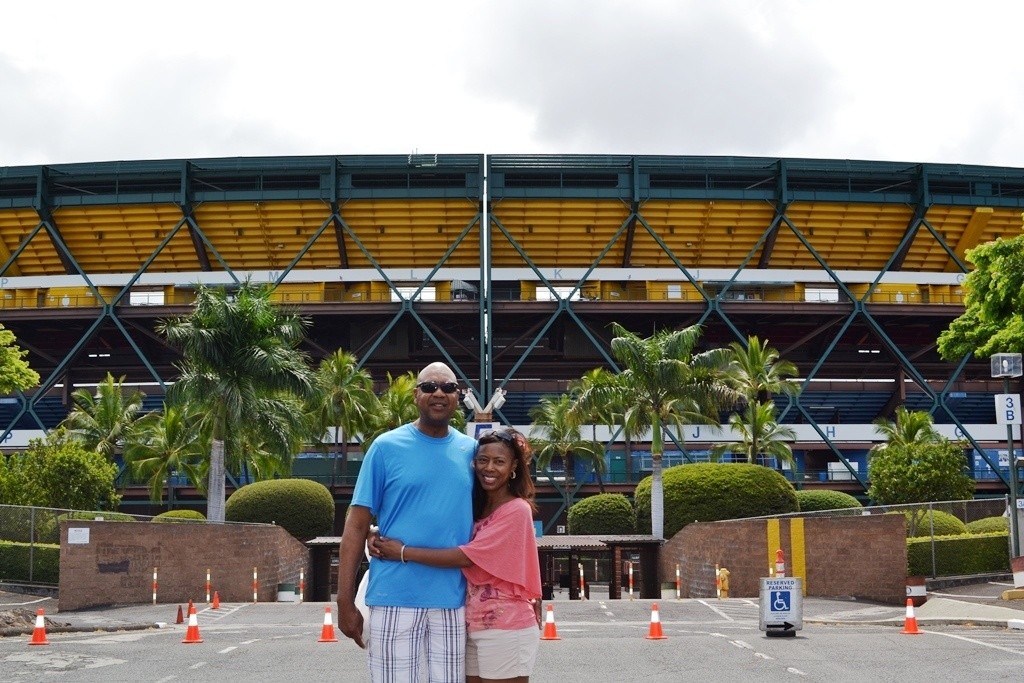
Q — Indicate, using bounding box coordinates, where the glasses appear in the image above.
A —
[477,429,514,447]
[415,381,460,393]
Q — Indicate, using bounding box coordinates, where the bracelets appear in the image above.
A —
[401,544,409,565]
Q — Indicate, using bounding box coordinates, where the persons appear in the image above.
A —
[337,361,481,683]
[368,427,543,683]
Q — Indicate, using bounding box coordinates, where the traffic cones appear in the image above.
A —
[539,604,563,640]
[316,605,340,642]
[211,591,222,609]
[26,608,48,645]
[643,604,668,639]
[181,606,204,642]
[899,599,926,635]
